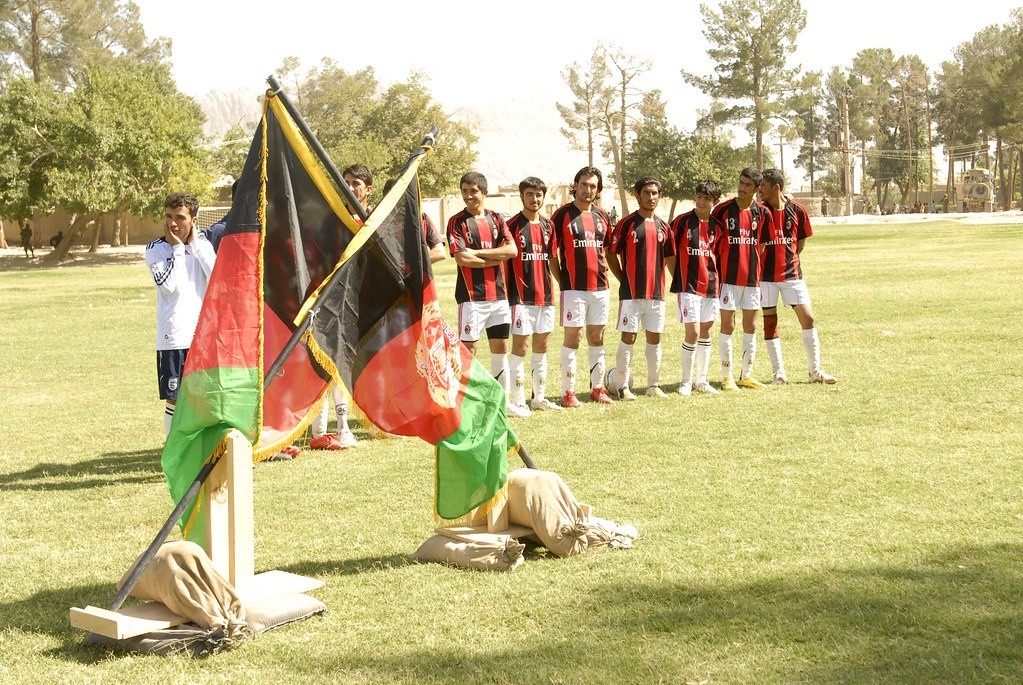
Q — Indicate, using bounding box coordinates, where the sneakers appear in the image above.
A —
[693,381,719,393]
[505,403,523,416]
[337,431,358,447]
[720,377,739,392]
[772,372,787,384]
[678,383,692,396]
[311,434,349,450]
[739,375,766,389]
[590,388,613,403]
[808,370,838,384]
[646,386,667,398]
[561,391,581,407]
[531,399,563,411]
[516,402,532,416]
[616,386,637,401]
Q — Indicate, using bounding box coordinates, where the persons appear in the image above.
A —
[201,178,300,459]
[343,165,402,439]
[50,231,64,248]
[606,178,676,401]
[712,166,778,389]
[821,197,829,217]
[963,196,967,208]
[668,178,726,396]
[145,192,216,434]
[309,386,357,451]
[383,179,447,264]
[552,167,613,405]
[761,168,837,384]
[20,223,34,257]
[447,172,518,415]
[862,194,949,214]
[509,179,562,414]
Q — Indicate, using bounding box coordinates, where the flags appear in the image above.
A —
[162,92,359,551]
[294,150,519,528]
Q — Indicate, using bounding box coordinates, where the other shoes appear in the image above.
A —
[282,446,300,457]
[269,452,291,461]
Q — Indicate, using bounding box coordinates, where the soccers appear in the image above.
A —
[604,366,634,395]
